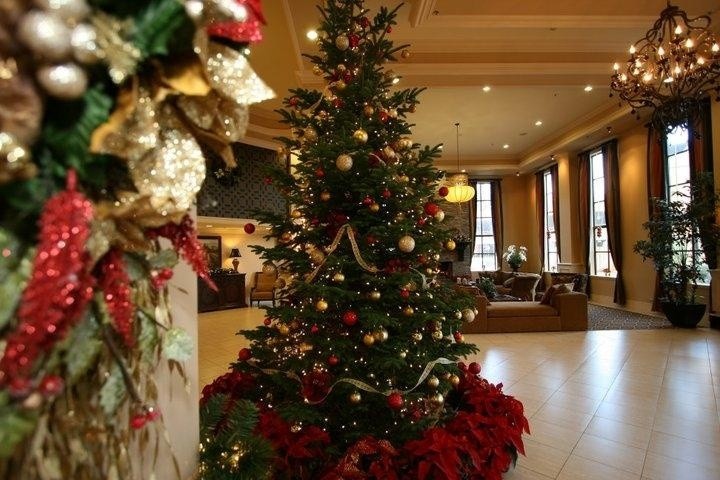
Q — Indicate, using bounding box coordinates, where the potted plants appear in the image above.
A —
[632,168,720,329]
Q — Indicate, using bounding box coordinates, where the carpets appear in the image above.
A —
[588,301,711,330]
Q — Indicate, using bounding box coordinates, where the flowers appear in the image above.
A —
[502,244,528,267]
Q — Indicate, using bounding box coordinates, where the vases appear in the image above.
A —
[511,265,519,272]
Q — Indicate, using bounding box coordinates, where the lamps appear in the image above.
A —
[444,123,476,206]
[229,249,242,273]
[608,0,720,144]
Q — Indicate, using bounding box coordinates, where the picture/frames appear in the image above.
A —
[197,235,222,269]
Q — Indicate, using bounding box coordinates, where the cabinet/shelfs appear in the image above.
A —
[198,271,248,313]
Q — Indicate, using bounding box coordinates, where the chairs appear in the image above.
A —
[250,271,278,307]
[452,272,589,334]
